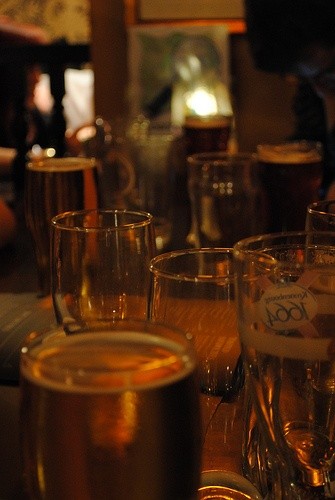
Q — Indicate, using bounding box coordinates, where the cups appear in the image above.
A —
[188,153,256,248]
[25,155,98,302]
[307,200,335,244]
[233,231,335,500]
[144,247,277,500]
[185,115,232,152]
[260,142,323,231]
[51,209,156,334]
[19,316,203,500]
